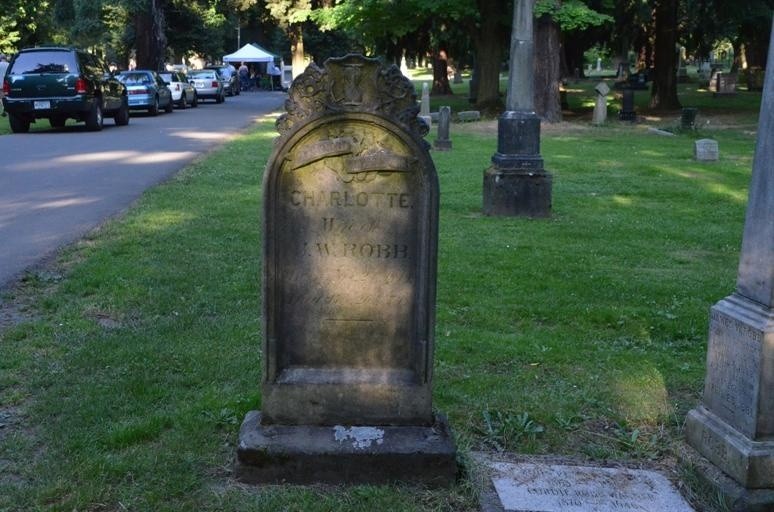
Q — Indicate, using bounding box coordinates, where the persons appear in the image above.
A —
[238,61,248,90]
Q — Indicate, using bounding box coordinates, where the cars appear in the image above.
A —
[0,43,241,133]
